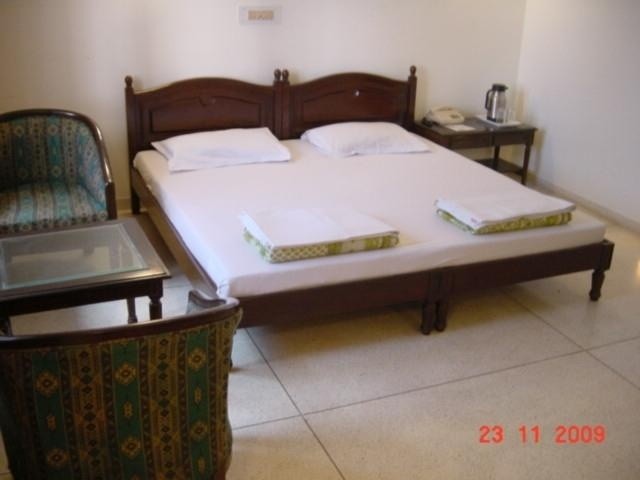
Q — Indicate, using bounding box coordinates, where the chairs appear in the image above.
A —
[1,108,118,239]
[0,290,244,479]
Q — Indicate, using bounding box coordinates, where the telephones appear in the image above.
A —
[424,105,465,126]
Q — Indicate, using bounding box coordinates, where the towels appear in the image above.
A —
[234,208,400,252]
[433,185,577,232]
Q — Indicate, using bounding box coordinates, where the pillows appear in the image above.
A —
[299,121,430,158]
[151,127,290,172]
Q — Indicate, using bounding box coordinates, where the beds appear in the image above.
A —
[281,69,615,333]
[126,66,442,337]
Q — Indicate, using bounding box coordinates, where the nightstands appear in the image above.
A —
[412,116,537,185]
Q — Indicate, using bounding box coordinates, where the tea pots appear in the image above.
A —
[485,83,509,122]
